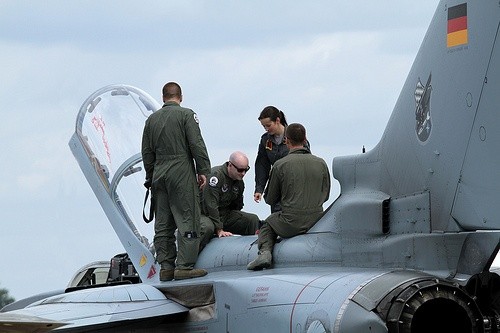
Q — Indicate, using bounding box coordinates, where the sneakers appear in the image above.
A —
[247,251,272,271]
[174,268,208,280]
[160,269,174,281]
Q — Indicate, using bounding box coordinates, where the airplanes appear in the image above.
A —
[0,0,500,333]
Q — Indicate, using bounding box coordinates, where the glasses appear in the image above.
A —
[229,161,250,172]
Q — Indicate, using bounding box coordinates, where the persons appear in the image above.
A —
[254,106,310,203]
[141,82,211,281]
[247,123,331,270]
[199,152,262,257]
